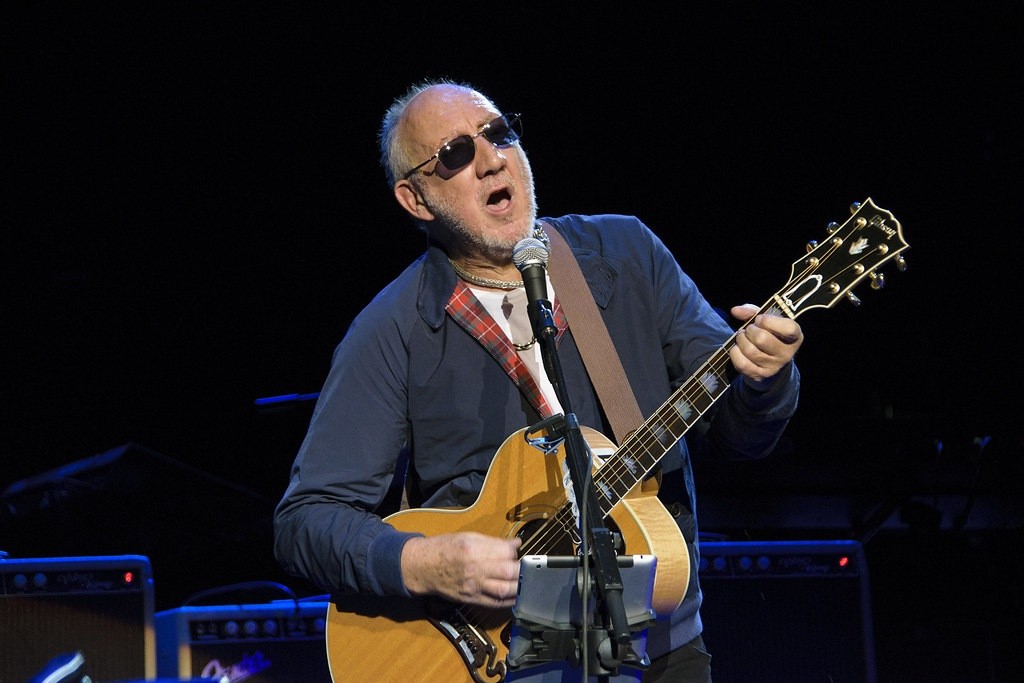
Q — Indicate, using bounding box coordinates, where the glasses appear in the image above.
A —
[402,112,524,178]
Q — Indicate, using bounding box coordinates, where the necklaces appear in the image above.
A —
[512,335,541,354]
[449,254,525,293]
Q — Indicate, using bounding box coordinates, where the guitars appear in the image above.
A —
[324,196,913,683]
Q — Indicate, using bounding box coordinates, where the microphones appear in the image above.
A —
[512,237,559,342]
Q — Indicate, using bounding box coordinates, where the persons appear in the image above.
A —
[272,81,803,683]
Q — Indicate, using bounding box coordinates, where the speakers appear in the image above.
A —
[700,539,878,683]
[1,553,158,683]
[155,602,332,683]
